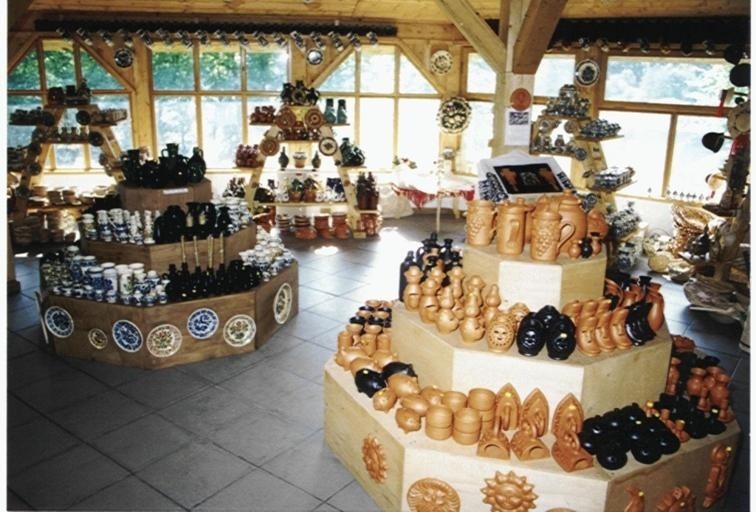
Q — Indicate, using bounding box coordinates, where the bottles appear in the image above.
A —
[83,207,159,243]
[465,199,574,263]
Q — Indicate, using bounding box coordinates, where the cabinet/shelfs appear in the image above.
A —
[40,178,297,368]
[222,80,382,241]
[8,82,124,251]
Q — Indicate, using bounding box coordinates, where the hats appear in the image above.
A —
[702,87,750,153]
[705,172,725,190]
[723,43,750,87]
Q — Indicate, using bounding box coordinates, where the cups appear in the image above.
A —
[42,253,171,305]
[240,232,292,280]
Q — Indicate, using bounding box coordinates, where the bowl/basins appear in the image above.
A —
[423,389,494,446]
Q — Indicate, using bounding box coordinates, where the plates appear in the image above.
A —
[113,47,134,69]
[434,96,473,136]
[44,283,294,361]
[430,49,454,76]
[259,109,338,157]
[573,59,602,88]
